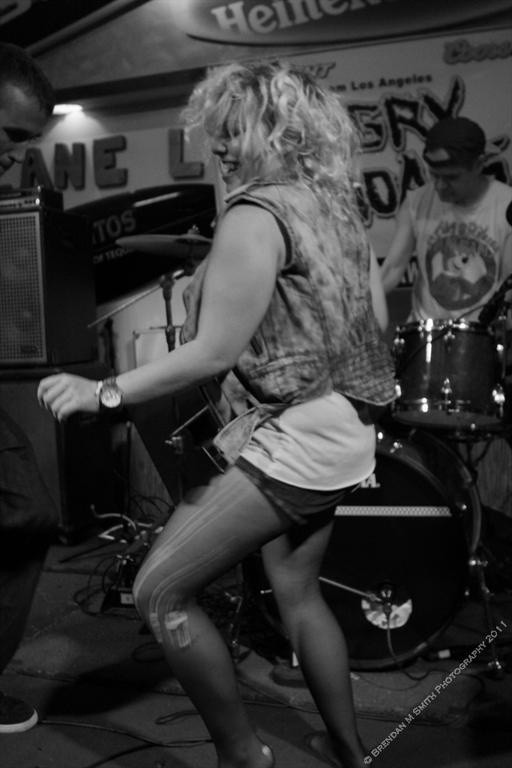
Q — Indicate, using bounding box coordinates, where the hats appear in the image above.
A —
[421,114,485,171]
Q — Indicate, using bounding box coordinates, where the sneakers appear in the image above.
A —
[0,689,40,732]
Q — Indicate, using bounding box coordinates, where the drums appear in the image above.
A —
[393,318,504,430]
[238,427,483,672]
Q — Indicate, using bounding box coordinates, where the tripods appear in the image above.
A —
[58,418,151,565]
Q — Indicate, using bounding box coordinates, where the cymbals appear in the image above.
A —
[115,234,213,250]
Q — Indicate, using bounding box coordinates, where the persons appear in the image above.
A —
[38,59,401,768]
[0,49,59,733]
[378,117,512,325]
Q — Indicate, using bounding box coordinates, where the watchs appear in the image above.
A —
[99,375,124,409]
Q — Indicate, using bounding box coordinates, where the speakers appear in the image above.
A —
[0,210,100,367]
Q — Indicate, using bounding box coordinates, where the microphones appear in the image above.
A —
[479,273,512,322]
